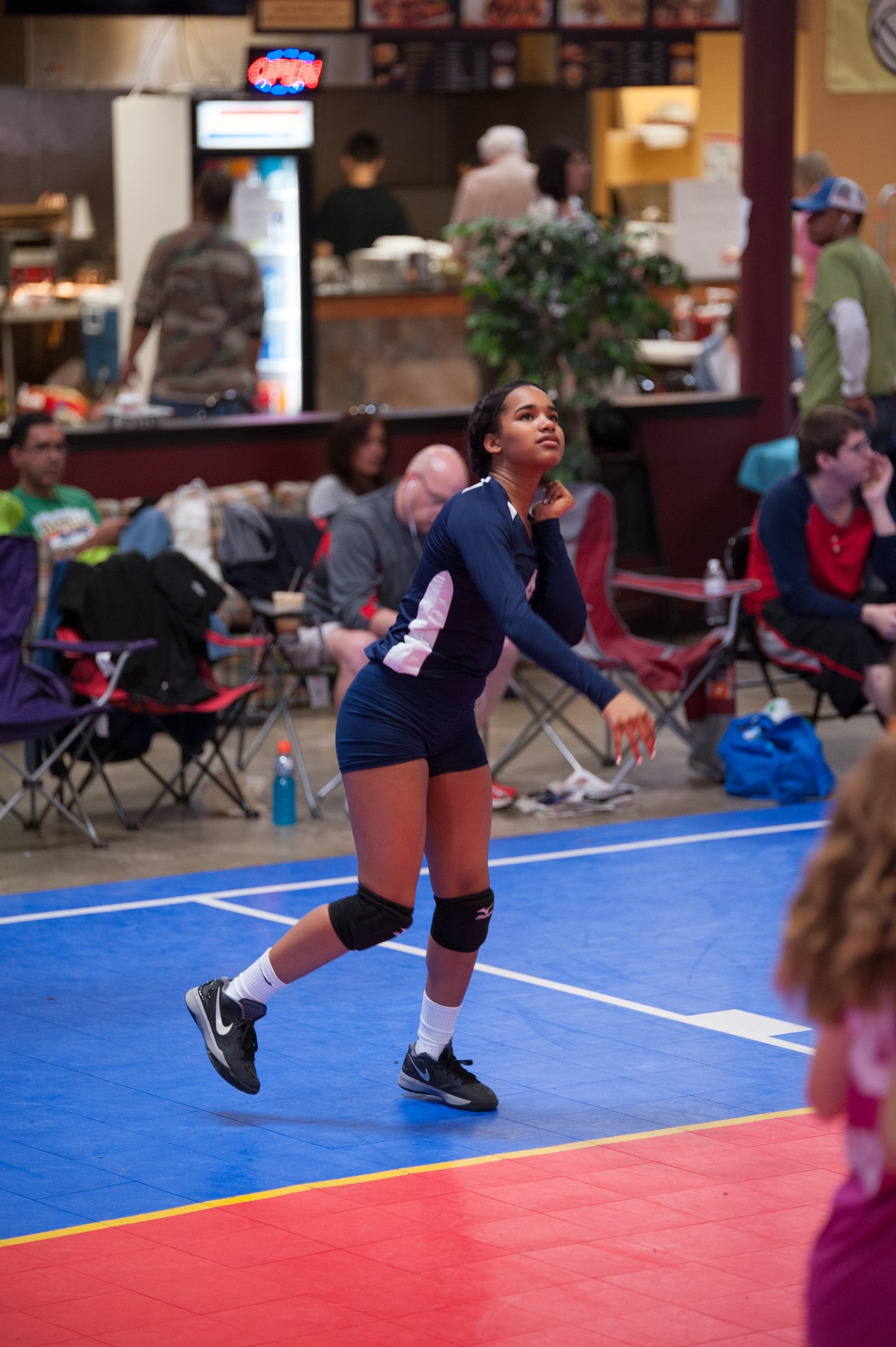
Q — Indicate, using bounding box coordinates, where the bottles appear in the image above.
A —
[272,740,295,826]
[704,559,728,625]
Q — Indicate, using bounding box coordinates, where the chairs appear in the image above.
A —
[479,480,761,811]
[21,549,274,833]
[208,480,296,713]
[211,500,344,824]
[722,524,890,730]
[0,534,159,852]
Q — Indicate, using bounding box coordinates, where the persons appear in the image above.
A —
[184,379,660,1112]
[778,722,896,1347]
[1,126,896,809]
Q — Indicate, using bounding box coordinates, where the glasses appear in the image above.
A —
[417,473,447,506]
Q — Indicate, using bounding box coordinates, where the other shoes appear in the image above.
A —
[493,779,516,809]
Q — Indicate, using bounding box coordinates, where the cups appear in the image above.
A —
[409,253,428,287]
[272,590,305,643]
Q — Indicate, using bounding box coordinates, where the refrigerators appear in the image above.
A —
[110,89,317,414]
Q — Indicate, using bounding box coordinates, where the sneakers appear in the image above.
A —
[185,975,268,1094]
[398,1038,498,1112]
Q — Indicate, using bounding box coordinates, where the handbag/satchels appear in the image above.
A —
[718,711,835,806]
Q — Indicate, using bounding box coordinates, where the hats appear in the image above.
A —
[792,175,869,214]
